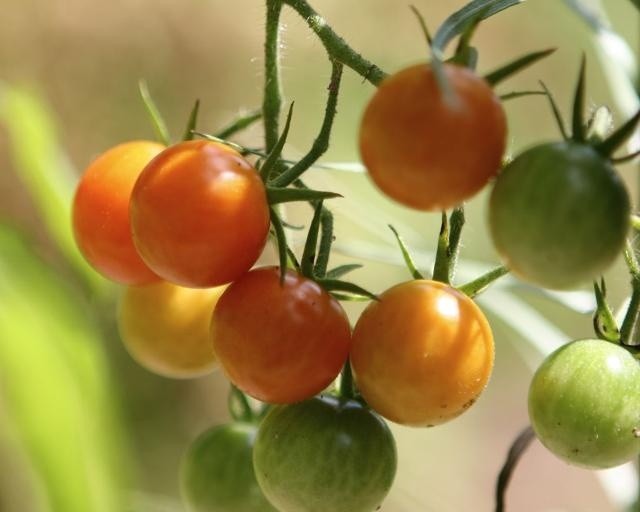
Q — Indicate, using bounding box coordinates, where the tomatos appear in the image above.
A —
[69,56,639,511]
